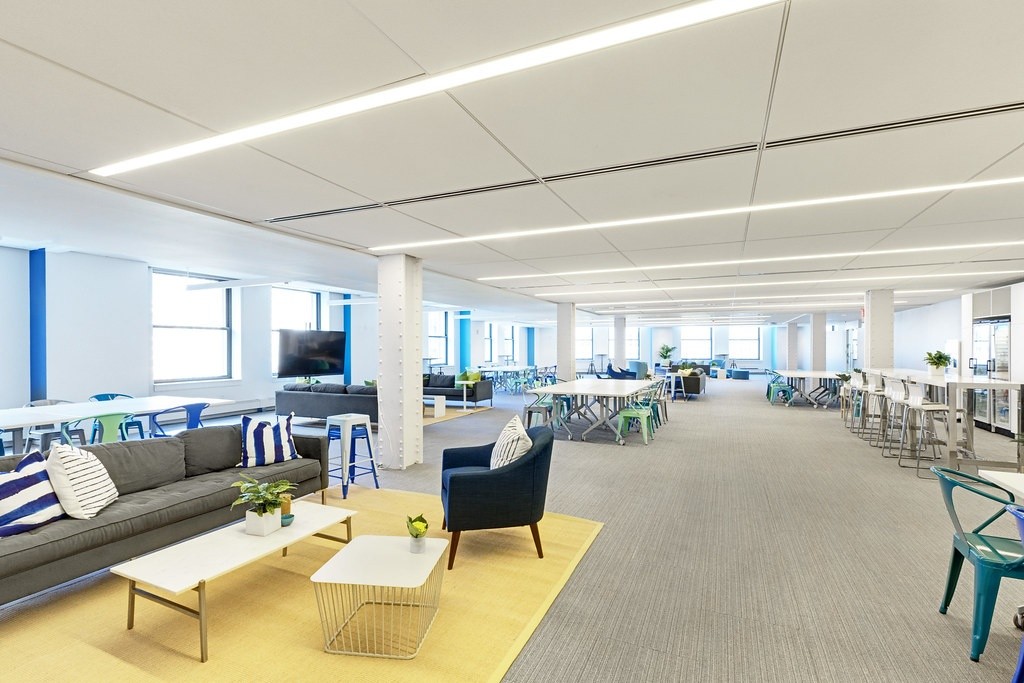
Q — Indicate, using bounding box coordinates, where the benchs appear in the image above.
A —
[0,424,330,606]
[275,379,494,425]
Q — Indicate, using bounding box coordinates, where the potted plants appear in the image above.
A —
[229,474,301,536]
[405,513,429,553]
[923,349,952,376]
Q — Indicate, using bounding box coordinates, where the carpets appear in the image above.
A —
[424,406,490,429]
[0,485,605,683]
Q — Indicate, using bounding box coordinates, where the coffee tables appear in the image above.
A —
[110,499,450,664]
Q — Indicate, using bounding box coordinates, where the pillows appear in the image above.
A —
[490,415,532,471]
[237,413,302,467]
[0,443,118,540]
[454,369,481,388]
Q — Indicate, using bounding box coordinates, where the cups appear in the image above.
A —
[281,491,291,514]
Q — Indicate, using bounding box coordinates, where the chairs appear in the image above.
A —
[480,362,670,444]
[763,369,793,407]
[0,391,212,456]
[931,467,1024,683]
[441,427,554,570]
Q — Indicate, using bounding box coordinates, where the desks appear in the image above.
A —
[0,397,238,454]
[861,366,1024,474]
[478,366,534,372]
[775,369,852,410]
[527,378,658,445]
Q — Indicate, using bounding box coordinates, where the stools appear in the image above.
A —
[838,372,979,480]
[322,413,379,500]
[660,373,688,403]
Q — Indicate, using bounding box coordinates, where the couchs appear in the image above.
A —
[677,368,707,394]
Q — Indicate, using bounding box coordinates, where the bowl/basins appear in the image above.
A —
[281,514,295,526]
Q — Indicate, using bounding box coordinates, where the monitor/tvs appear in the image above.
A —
[277,329,346,378]
